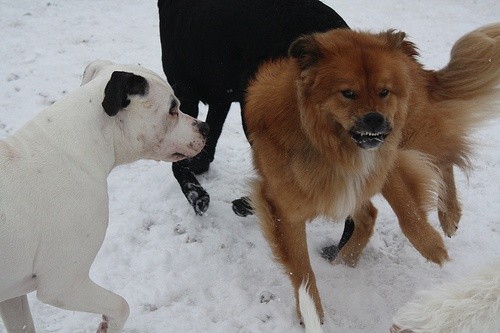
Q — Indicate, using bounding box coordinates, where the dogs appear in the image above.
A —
[1,59,209,332]
[157,0,355,261]
[243,21,500,333]
